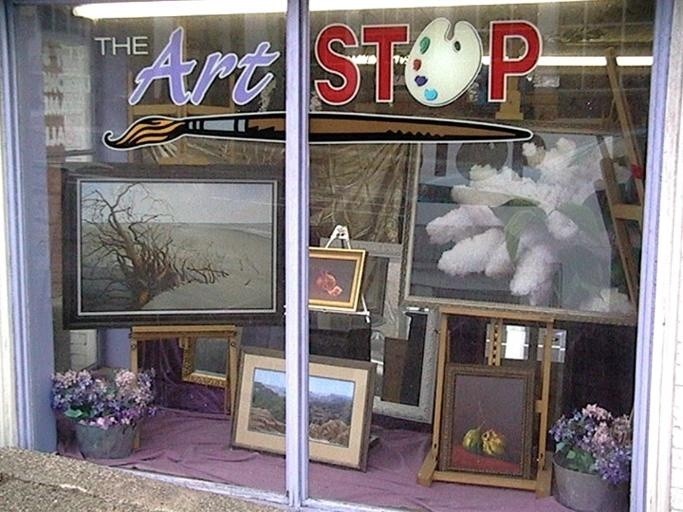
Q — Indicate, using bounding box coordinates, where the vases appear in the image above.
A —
[71,422,139,459]
[553,455,629,511]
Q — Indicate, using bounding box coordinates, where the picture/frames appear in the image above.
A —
[317,237,410,376]
[181,337,230,388]
[372,300,438,425]
[229,344,378,474]
[60,161,287,331]
[308,247,367,312]
[397,117,642,328]
[439,362,536,480]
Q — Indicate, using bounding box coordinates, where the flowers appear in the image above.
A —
[549,403,633,485]
[48,367,157,430]
